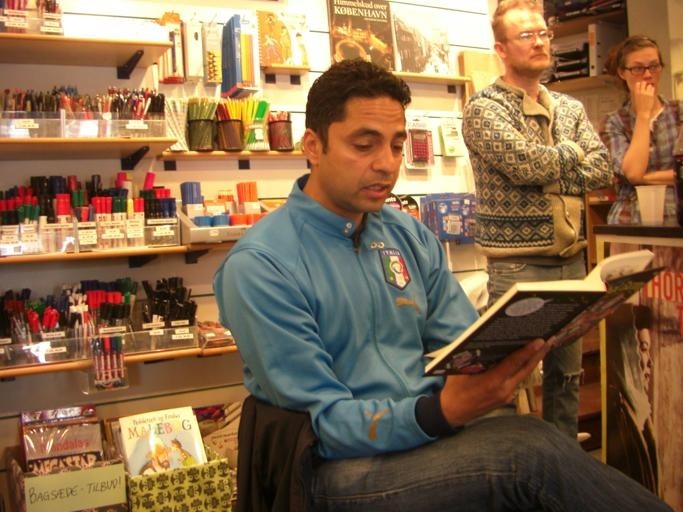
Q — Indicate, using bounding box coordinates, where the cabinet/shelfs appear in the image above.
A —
[0,32,310,377]
[535,0,627,94]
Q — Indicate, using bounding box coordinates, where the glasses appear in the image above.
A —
[625,62,663,76]
[505,29,555,43]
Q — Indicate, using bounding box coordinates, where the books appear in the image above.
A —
[421,249,666,379]
[325,0,395,71]
[389,12,451,75]
[541,0,627,84]
[19,400,243,477]
[255,11,312,72]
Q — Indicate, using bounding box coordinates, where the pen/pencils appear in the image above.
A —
[91,334,127,389]
[0,84,292,152]
[0,172,177,252]
[0,277,198,364]
[0,0,61,34]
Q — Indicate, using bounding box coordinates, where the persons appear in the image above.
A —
[598,32,682,231]
[456,0,613,443]
[210,58,672,512]
[604,302,661,497]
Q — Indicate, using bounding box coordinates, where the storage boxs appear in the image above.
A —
[10,445,233,512]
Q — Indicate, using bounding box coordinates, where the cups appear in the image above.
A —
[189,117,293,153]
[634,183,668,226]
